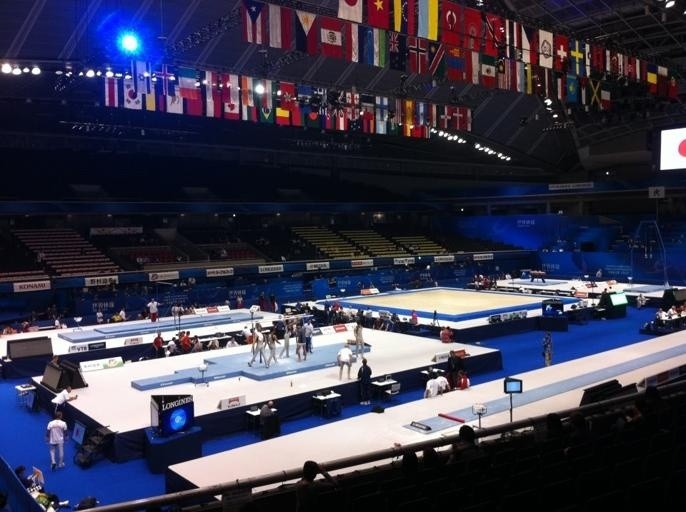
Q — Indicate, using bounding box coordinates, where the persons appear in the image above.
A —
[293,461,337,511]
[634,293,686,330]
[94,262,205,359]
[346,305,419,333]
[337,321,372,405]
[0,302,73,335]
[48,384,77,420]
[432,310,453,343]
[541,329,552,368]
[258,400,274,437]
[14,465,46,494]
[442,384,665,476]
[44,411,69,471]
[470,265,615,314]
[423,351,470,400]
[206,291,344,368]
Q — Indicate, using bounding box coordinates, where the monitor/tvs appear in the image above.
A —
[504,379,522,393]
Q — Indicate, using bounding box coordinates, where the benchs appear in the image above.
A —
[182,394,686,512]
[0,213,524,283]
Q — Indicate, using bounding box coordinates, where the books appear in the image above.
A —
[97,58,472,138]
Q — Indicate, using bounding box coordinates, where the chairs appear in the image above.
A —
[384,383,401,404]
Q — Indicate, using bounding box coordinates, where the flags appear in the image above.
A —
[660,127,686,172]
[242,0,684,107]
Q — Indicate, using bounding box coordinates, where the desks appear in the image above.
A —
[311,390,342,417]
[371,379,398,400]
[15,384,37,409]
[420,368,446,387]
